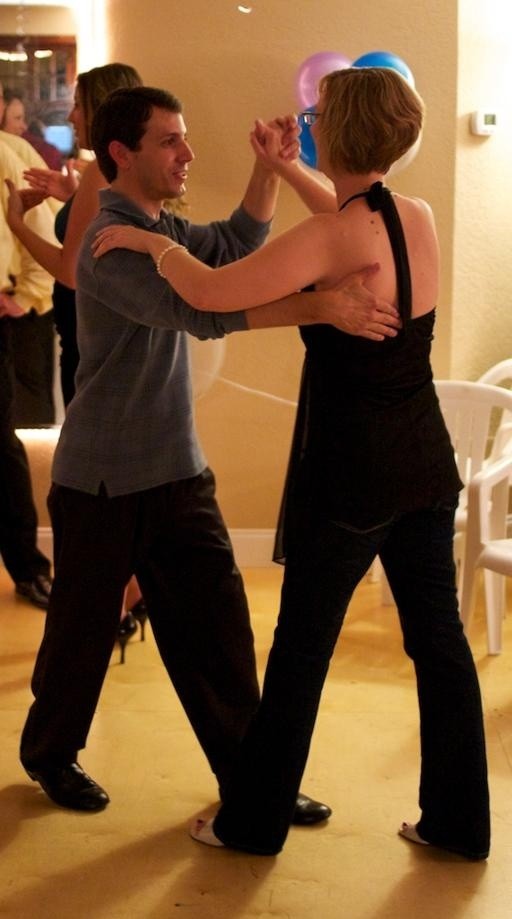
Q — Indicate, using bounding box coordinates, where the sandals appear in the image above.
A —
[190,818,225,847]
[399,823,429,845]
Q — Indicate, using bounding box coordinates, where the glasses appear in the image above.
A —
[302,111,320,126]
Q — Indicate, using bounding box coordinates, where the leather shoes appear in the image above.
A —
[293,793,331,824]
[16,575,53,609]
[25,762,108,810]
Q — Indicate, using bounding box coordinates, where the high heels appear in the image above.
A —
[118,598,147,663]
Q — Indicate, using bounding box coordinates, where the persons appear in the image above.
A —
[84,63,494,866]
[12,83,411,842]
[2,82,77,615]
[5,63,149,671]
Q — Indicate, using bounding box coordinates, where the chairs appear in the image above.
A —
[431,359,512,657]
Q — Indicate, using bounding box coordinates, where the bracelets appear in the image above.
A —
[155,242,188,281]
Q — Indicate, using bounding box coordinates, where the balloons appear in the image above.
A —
[288,49,424,181]
